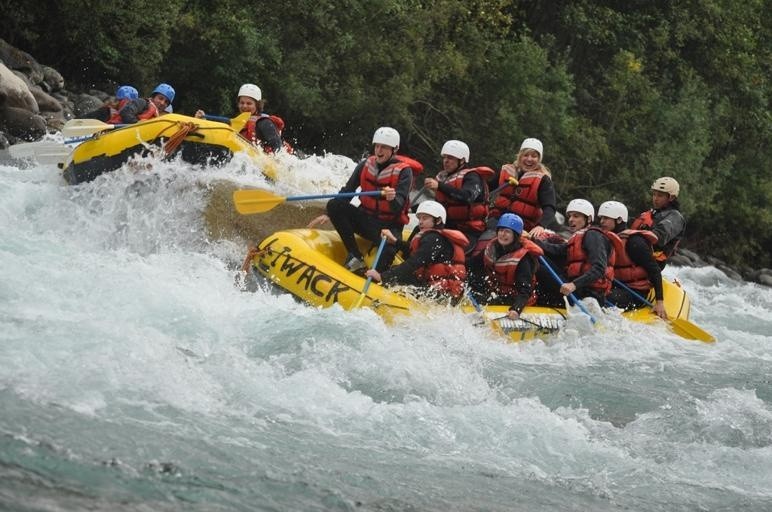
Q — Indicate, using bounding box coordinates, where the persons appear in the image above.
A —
[597,201,669,321]
[366,200,469,306]
[521,198,622,311]
[195,84,285,154]
[263,114,292,155]
[85,85,140,121]
[629,177,688,273]
[110,84,179,123]
[472,137,556,259]
[472,212,545,319]
[409,138,494,255]
[308,126,423,274]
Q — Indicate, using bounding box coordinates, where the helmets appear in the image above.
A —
[440,139,470,164]
[372,126,400,153]
[115,85,139,102]
[520,137,543,162]
[495,212,524,234]
[597,200,629,223]
[152,83,176,104]
[416,200,447,224]
[651,176,680,199]
[566,198,595,222]
[237,83,262,102]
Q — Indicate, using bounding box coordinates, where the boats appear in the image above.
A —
[239,228,692,344]
[60,112,344,194]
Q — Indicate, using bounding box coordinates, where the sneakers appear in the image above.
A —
[343,256,368,274]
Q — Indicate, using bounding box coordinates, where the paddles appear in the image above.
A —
[349,234,386,310]
[63,119,135,137]
[613,277,715,344]
[202,112,251,133]
[464,289,509,343]
[234,190,386,215]
